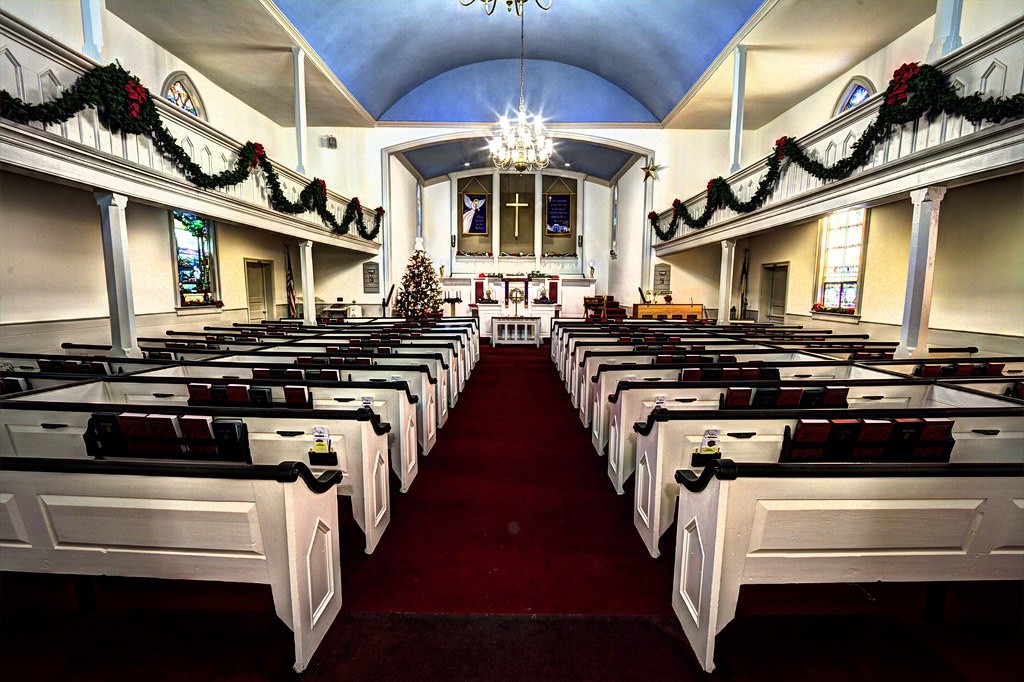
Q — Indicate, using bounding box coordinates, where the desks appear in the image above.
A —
[491,316,540,348]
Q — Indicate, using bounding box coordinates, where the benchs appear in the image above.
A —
[544,315,1024,674]
[2,310,482,682]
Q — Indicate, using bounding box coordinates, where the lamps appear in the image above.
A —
[459,0,556,17]
[477,3,553,174]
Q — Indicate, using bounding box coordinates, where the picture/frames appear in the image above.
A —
[461,192,489,236]
[545,193,573,236]
